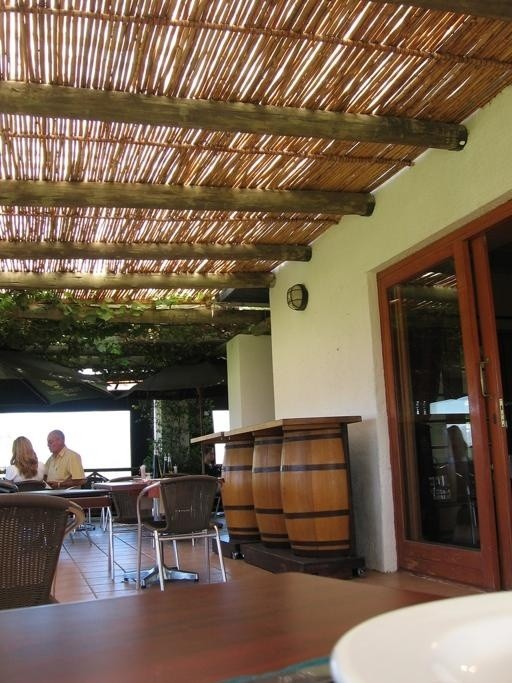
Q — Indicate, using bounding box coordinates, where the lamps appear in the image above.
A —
[287,284,308,311]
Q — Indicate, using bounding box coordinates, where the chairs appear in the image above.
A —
[0,471,226,611]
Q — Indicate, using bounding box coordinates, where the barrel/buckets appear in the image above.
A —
[416,422,457,542]
[221,429,353,557]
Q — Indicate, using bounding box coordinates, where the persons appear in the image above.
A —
[4,436,49,486]
[41,430,89,495]
[199,448,219,476]
[447,422,471,495]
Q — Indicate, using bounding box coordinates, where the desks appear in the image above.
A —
[0,569,456,683]
[188,414,362,580]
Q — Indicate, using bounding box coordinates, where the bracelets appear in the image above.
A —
[57,481,60,488]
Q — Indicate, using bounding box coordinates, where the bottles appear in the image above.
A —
[163,453,173,475]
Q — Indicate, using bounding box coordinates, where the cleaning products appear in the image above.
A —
[145,473,150,480]
